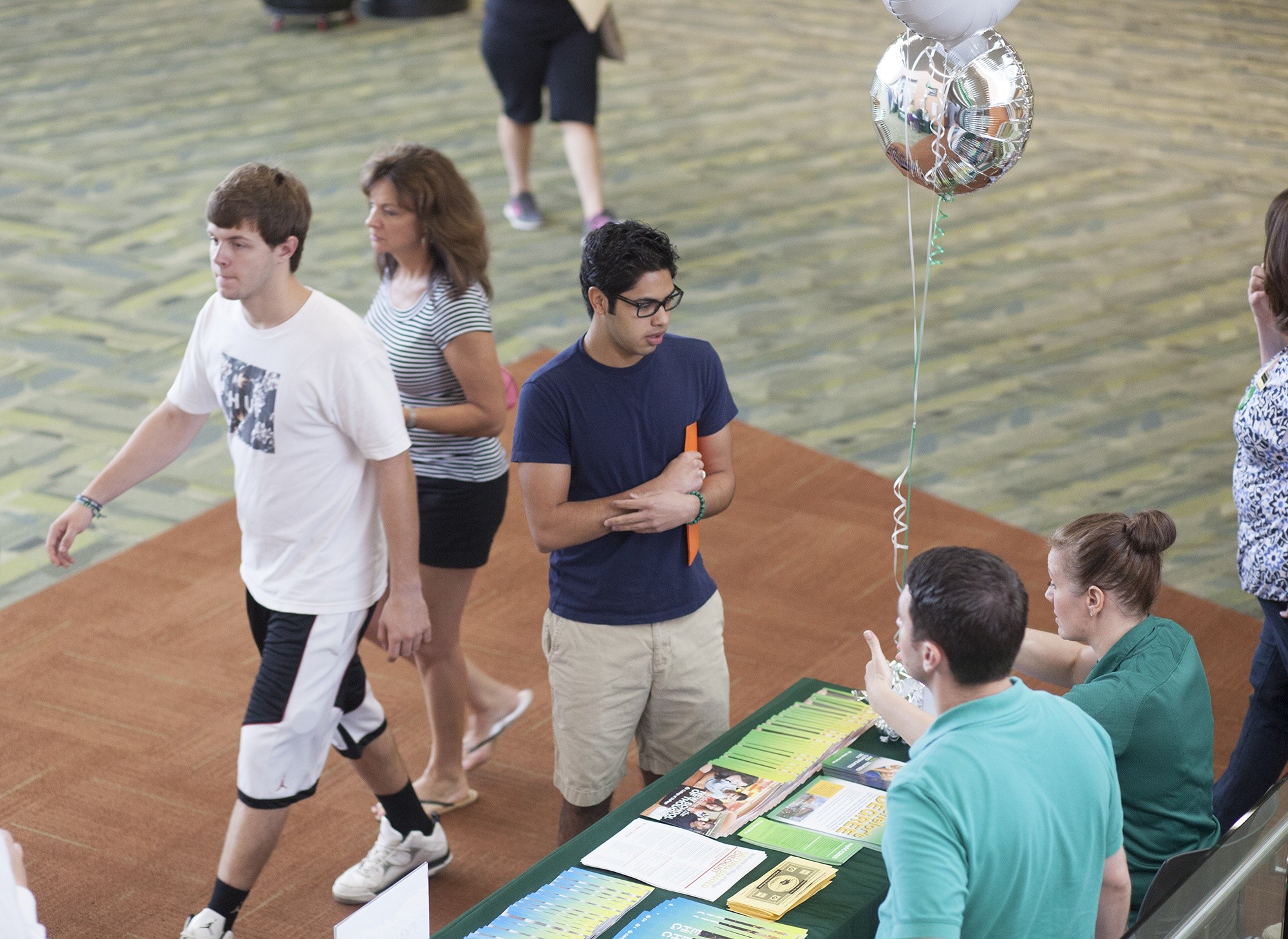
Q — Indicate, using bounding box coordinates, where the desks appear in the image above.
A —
[427,674,917,937]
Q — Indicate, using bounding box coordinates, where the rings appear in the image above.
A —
[701,468,707,480]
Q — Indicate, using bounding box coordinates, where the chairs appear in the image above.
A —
[1133,788,1276,920]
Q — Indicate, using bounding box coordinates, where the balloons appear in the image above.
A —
[885,2,1023,43]
[870,22,1036,201]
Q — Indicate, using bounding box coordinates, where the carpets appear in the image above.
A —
[0,344,1288,938]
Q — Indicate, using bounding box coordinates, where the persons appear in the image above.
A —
[1202,184,1288,820]
[656,766,759,833]
[870,545,1134,939]
[859,508,1221,923]
[507,219,736,843]
[360,140,540,820]
[2,829,47,939]
[477,1,626,245]
[41,164,461,939]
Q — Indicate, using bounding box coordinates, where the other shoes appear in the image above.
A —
[502,190,544,231]
[580,208,620,245]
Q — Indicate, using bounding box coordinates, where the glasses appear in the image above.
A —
[615,283,684,318]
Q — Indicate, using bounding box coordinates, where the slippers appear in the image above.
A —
[461,688,534,754]
[374,787,480,821]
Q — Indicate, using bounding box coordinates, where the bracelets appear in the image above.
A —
[401,402,418,429]
[71,493,108,520]
[686,491,706,526]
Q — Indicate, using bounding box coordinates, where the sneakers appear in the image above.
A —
[179,909,234,939]
[330,814,453,906]
